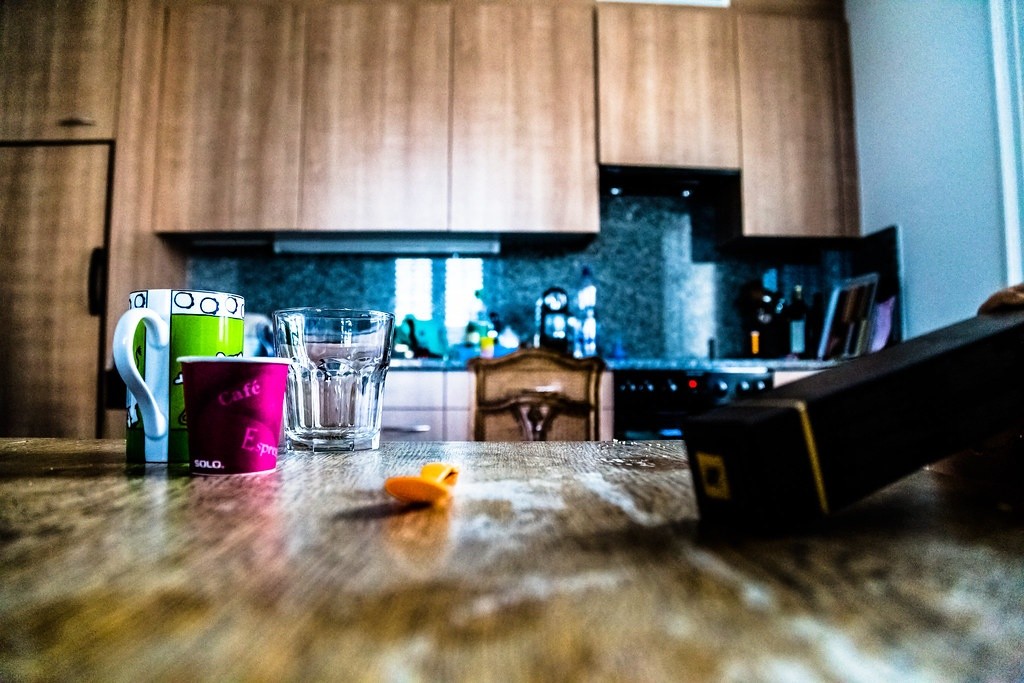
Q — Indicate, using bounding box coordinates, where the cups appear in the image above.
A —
[271,307,396,451]
[177,356,294,478]
[112,288,246,469]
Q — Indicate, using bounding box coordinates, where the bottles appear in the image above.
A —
[573,266,598,358]
[466,289,488,347]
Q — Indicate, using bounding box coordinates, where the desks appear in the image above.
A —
[0,435,1024,683]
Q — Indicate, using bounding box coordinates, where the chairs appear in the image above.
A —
[468,348,607,441]
